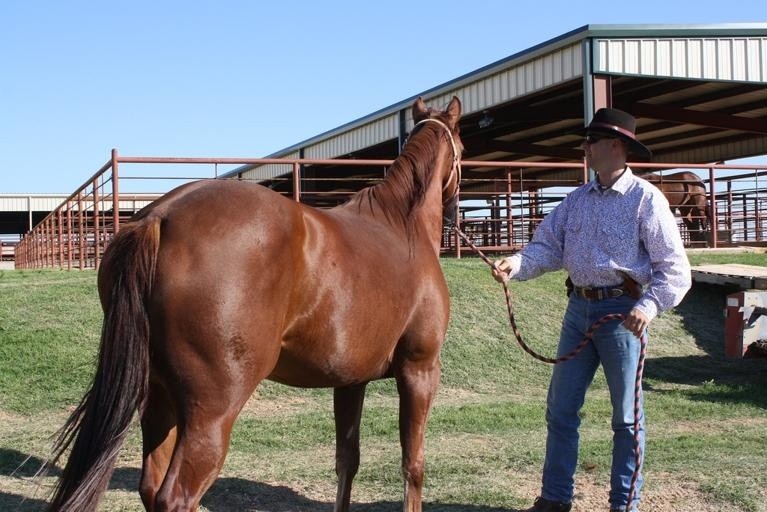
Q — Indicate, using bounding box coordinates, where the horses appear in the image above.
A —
[634,171,712,248]
[7,95,468,512]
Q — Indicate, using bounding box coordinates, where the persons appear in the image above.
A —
[489,106,695,510]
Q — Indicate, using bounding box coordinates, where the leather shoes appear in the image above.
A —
[516,496,573,512]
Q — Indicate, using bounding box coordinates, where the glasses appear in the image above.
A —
[584,131,619,145]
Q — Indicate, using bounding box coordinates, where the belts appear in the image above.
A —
[567,279,637,301]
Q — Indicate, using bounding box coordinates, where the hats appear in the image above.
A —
[569,106,653,173]
